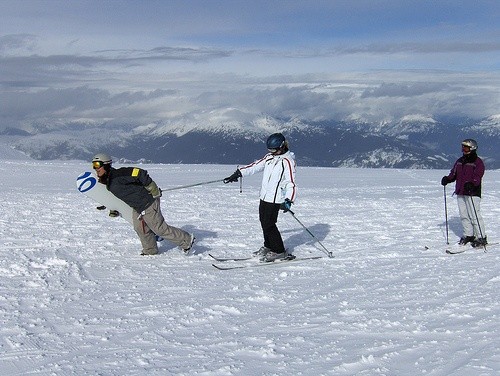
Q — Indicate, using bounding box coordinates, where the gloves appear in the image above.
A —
[153,191,162,199]
[441,176,452,186]
[223,169,242,185]
[464,181,476,192]
[281,198,293,213]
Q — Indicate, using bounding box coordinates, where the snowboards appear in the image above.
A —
[76,171,165,243]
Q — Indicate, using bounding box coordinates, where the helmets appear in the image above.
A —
[461,139,478,154]
[266,132,285,153]
[92,153,112,165]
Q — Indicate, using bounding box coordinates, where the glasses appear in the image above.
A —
[92,161,108,169]
[462,146,472,152]
[268,148,280,153]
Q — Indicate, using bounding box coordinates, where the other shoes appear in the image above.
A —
[183,233,198,252]
[140,251,160,257]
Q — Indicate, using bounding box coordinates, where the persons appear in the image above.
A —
[91,153,196,255]
[229,133,297,262]
[442,139,488,247]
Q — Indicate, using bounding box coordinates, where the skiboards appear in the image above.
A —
[208,250,322,270]
[425,237,499,254]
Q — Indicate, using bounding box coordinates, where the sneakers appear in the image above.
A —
[471,235,488,247]
[259,250,289,263]
[253,247,280,257]
[460,236,477,246]
